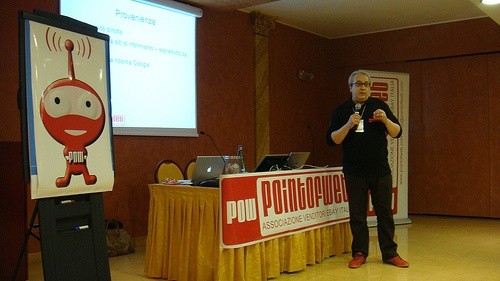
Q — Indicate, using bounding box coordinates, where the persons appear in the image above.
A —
[325,69,411,270]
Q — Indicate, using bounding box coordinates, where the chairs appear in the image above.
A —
[185,159,196,179]
[154,159,185,183]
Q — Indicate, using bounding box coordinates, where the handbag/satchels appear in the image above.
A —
[104,220,134,257]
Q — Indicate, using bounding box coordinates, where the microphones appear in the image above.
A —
[353,103,361,130]
[199,130,226,164]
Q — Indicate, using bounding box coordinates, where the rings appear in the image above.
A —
[379,113,383,116]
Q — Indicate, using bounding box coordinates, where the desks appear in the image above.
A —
[144,179,352,281]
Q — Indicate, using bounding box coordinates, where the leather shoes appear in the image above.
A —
[348,256,366,268]
[382,255,409,266]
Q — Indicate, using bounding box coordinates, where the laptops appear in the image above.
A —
[176,155,225,185]
[250,155,289,172]
[282,153,311,169]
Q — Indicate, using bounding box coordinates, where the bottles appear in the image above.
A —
[237,145,246,175]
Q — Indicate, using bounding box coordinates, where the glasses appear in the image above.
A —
[351,81,371,88]
